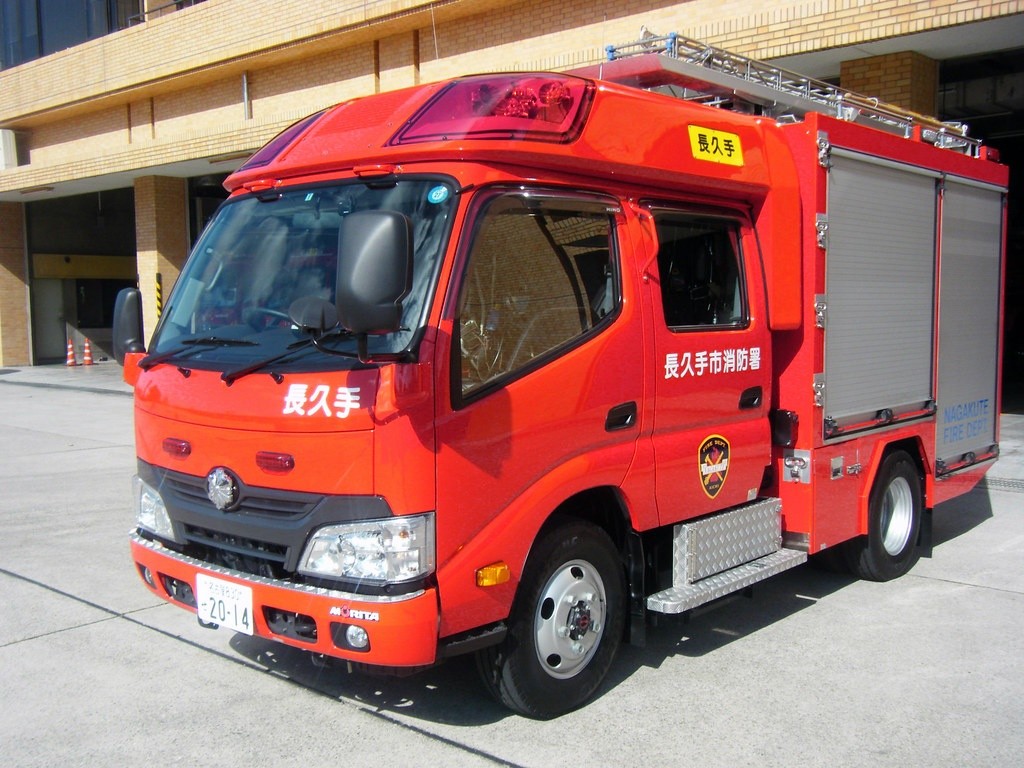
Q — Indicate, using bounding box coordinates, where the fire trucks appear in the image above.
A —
[110,32,1012,724]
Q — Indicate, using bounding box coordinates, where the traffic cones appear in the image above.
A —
[65,338,81,367]
[81,337,99,365]
